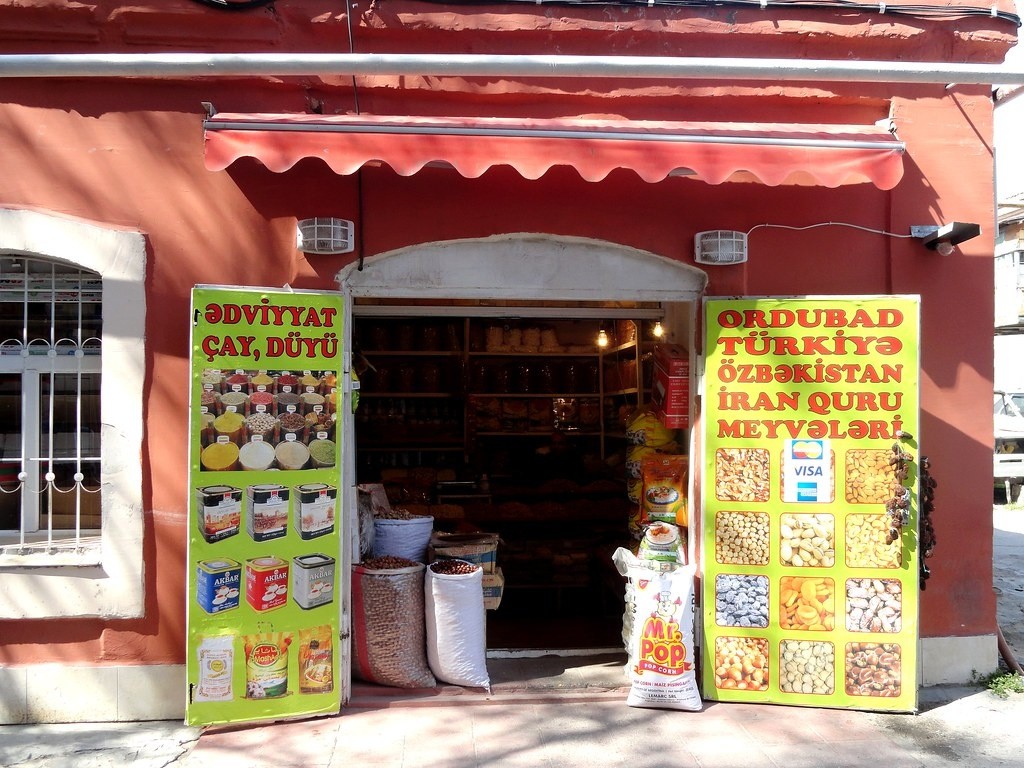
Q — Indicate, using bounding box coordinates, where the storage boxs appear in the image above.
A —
[427,531,499,573]
[41,485,101,529]
[650,344,689,430]
[482,567,505,610]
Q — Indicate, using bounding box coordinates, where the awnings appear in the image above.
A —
[203,109,907,191]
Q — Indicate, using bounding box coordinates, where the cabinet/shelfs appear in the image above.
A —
[354,318,644,595]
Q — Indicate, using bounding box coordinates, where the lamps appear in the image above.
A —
[294,217,354,254]
[693,230,748,265]
[923,221,981,256]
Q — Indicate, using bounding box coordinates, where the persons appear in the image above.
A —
[535,432,576,487]
